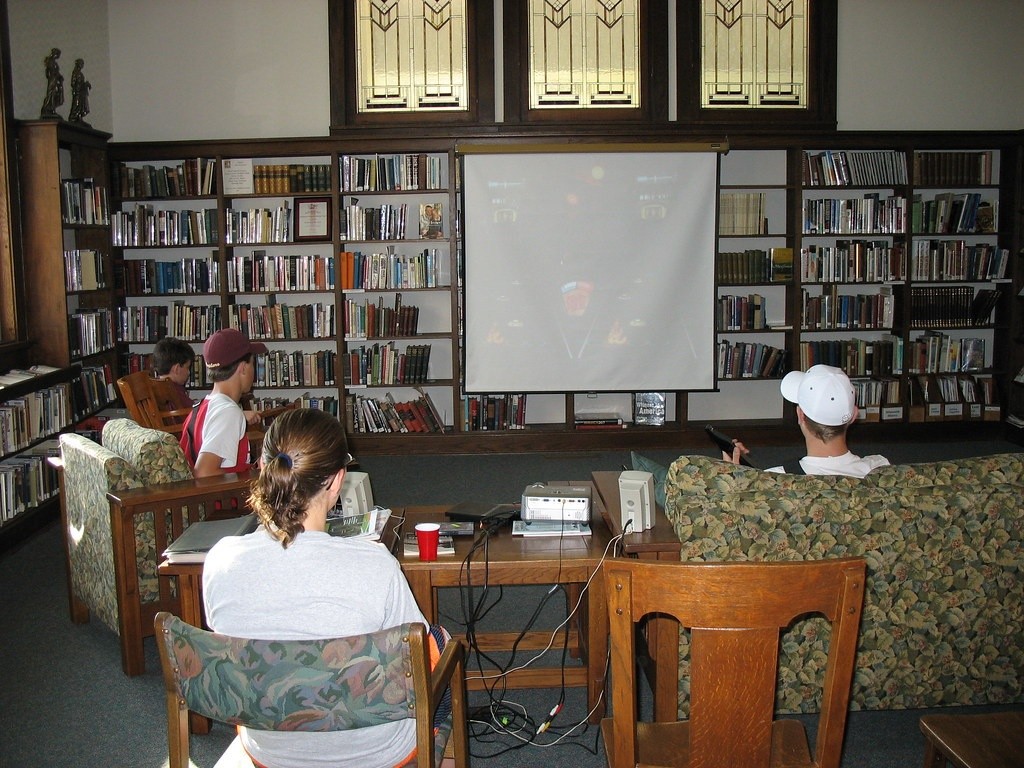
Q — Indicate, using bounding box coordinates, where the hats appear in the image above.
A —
[204,329,267,370]
[781,364,856,426]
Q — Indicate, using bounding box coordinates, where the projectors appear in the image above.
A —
[521,484,593,527]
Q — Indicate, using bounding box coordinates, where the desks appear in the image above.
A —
[394,505,623,725]
[158,508,404,734]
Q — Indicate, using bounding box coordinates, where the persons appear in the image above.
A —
[722,364,890,478]
[202,408,453,768]
[152,337,263,426]
[180,328,266,510]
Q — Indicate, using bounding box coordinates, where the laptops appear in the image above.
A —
[444,502,522,526]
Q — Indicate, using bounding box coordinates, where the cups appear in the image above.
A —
[415,523,440,561]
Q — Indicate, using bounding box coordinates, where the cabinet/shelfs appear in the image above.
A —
[0,364,82,557]
[457,133,1023,447]
[109,136,461,434]
[16,119,119,409]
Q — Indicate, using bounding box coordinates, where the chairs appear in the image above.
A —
[117,370,295,462]
[919,711,1023,768]
[155,611,470,768]
[46,418,261,678]
[601,557,867,768]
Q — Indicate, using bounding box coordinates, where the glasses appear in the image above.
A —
[326,452,354,491]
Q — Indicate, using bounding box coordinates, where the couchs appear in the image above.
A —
[569,455,1024,719]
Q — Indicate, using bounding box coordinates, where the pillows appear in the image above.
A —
[630,451,668,509]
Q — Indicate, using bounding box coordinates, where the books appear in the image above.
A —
[632,392,667,426]
[800,150,1009,407]
[326,508,392,540]
[0,383,66,527]
[460,393,526,431]
[404,521,474,556]
[575,413,628,430]
[165,514,260,564]
[112,152,445,433]
[718,193,793,378]
[60,178,118,423]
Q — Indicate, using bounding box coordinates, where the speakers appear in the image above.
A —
[618,471,656,532]
[339,471,374,517]
